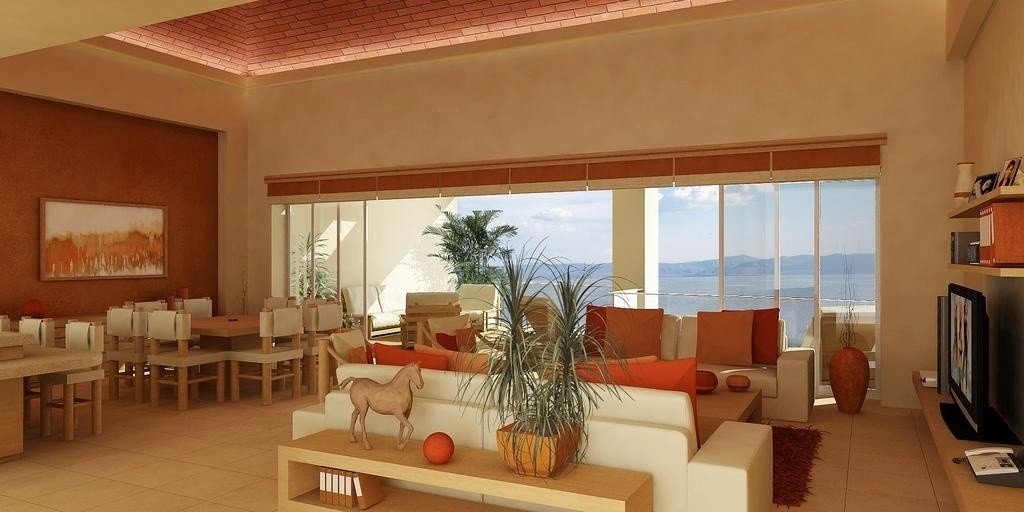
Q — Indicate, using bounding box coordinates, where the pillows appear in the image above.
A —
[604,307,665,357]
[752,307,779,363]
[697,311,755,368]
[582,303,605,359]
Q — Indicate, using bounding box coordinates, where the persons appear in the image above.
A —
[952,295,967,395]
[997,160,1015,186]
[971,176,994,199]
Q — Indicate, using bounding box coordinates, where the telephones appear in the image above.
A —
[952,446,1024,489]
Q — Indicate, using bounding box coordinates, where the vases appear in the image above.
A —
[829,347,870,412]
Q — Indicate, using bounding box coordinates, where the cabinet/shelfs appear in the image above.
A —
[278,428,653,512]
[943,187,1024,278]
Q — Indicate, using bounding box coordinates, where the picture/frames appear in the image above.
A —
[37,194,168,285]
[968,174,996,200]
[995,157,1022,190]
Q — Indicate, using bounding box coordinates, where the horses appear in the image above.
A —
[328,360,424,450]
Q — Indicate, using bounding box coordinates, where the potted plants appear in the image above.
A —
[453,234,644,480]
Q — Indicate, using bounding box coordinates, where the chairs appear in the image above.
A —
[457,283,500,332]
[400,292,460,348]
[343,283,406,336]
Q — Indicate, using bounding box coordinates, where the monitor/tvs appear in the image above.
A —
[939,282,1024,446]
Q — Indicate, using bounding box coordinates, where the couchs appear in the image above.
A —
[579,304,814,422]
[291,361,774,512]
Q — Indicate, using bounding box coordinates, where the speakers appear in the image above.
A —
[950,232,980,265]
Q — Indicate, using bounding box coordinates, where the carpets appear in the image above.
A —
[758,418,830,511]
